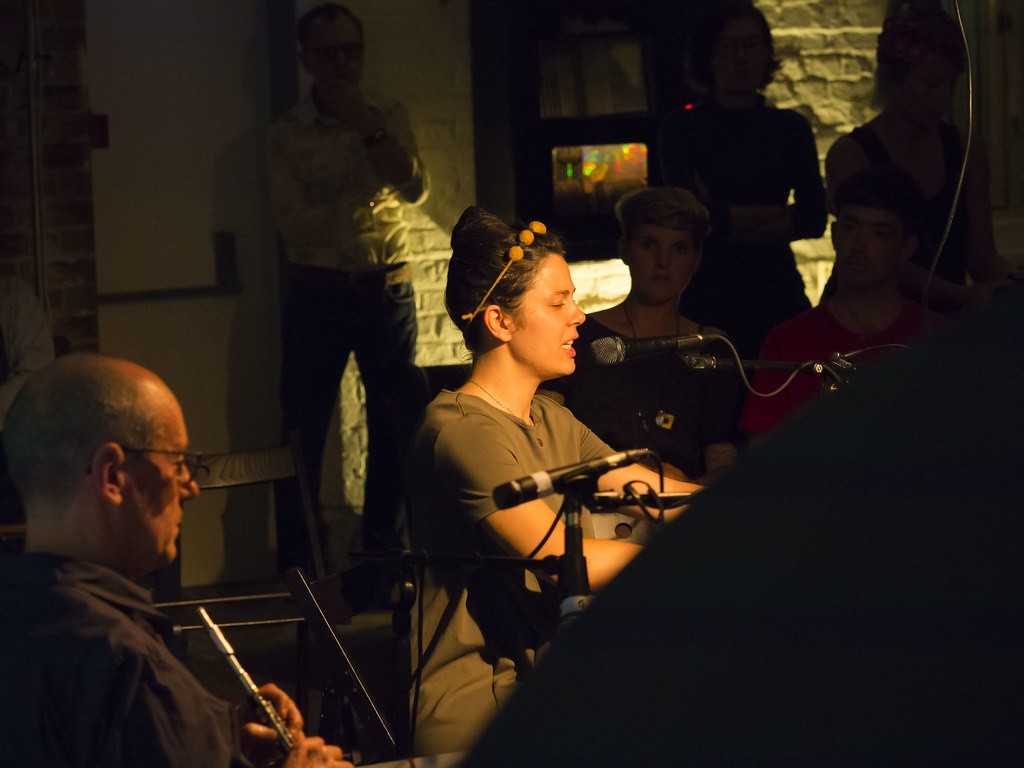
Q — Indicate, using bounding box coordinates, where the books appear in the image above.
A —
[539,31,647,118]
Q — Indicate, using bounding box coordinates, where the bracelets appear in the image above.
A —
[362,129,387,147]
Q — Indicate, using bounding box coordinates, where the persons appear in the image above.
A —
[658,0,827,361]
[405,204,706,757]
[825,7,1015,323]
[0,351,356,768]
[535,186,738,486]
[270,3,425,612]
[741,165,963,451]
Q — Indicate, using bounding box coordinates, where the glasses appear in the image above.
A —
[713,35,767,56]
[85,443,206,487]
[304,42,361,63]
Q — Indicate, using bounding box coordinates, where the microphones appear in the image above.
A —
[557,497,593,623]
[589,333,723,368]
[492,449,649,510]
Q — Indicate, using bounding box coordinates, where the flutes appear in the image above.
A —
[194,604,297,752]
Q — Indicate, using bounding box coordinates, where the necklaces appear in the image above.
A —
[623,304,679,431]
[469,380,542,447]
